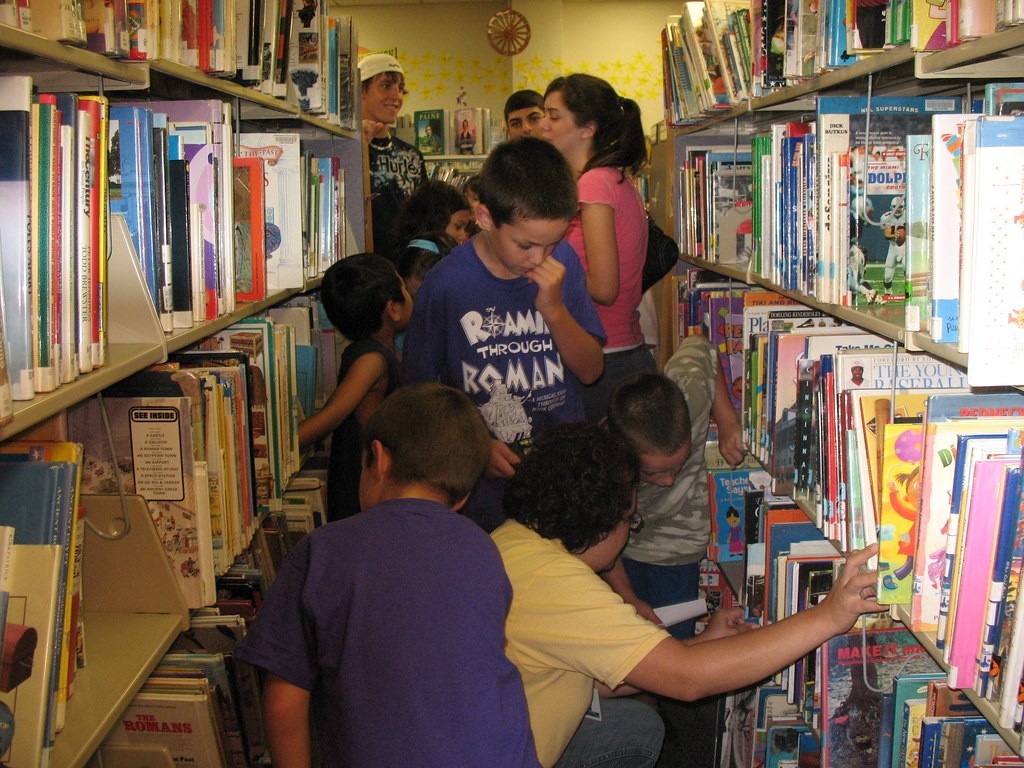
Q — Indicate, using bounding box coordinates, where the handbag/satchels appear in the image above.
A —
[642,211,678,296]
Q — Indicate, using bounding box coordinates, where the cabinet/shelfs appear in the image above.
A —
[0,23,374,768]
[658,27,1024,768]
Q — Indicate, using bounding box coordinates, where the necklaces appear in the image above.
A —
[369,131,392,151]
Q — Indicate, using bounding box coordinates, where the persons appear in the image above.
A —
[234,137,892,766]
[460,120,474,156]
[424,125,441,152]
[507,72,681,429]
[356,53,426,248]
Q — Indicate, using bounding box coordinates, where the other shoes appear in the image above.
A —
[868,290,877,304]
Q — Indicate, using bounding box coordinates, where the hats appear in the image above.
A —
[850,359,864,369]
[357,53,404,82]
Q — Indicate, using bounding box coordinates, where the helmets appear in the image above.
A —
[851,196,873,227]
[890,197,904,218]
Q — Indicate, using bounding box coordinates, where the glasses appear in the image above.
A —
[614,510,645,535]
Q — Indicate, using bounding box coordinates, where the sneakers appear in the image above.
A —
[885,285,892,296]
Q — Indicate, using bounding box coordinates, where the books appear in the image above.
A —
[667,2,1023,768]
[391,106,507,192]
[1,0,359,766]
